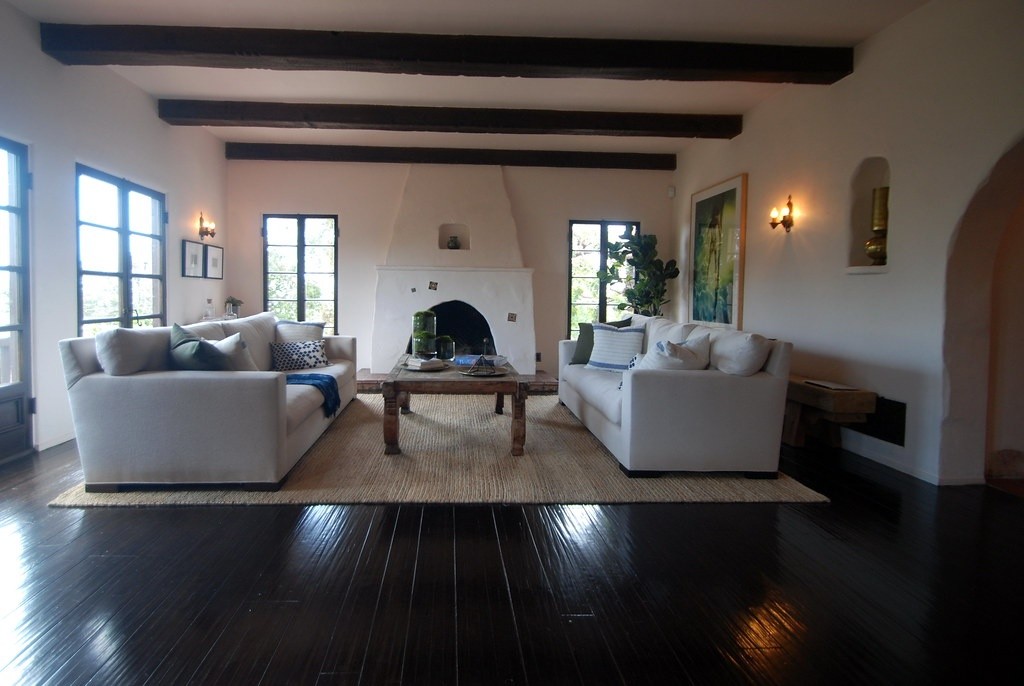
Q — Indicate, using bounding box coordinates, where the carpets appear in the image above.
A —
[48,395,830,506]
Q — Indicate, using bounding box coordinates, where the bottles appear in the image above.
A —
[222,303,237,320]
[202,299,216,320]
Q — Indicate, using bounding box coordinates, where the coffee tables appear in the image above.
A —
[382,356,530,455]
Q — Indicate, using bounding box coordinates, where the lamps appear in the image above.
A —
[770,195,793,232]
[198,212,216,240]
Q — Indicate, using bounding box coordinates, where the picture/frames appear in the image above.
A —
[182,240,204,277]
[687,170,749,330]
[205,245,224,279]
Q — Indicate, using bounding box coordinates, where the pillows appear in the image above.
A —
[201,333,260,373]
[618,352,646,390]
[270,339,327,369]
[168,323,236,372]
[277,321,327,343]
[640,330,710,369]
[569,317,632,365]
[585,322,646,372]
[711,332,773,377]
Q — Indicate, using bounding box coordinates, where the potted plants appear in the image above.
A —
[224,297,240,318]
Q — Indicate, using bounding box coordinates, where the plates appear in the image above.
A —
[456,366,511,376]
[402,364,450,372]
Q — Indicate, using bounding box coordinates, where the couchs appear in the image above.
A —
[62,311,357,492]
[557,313,792,477]
